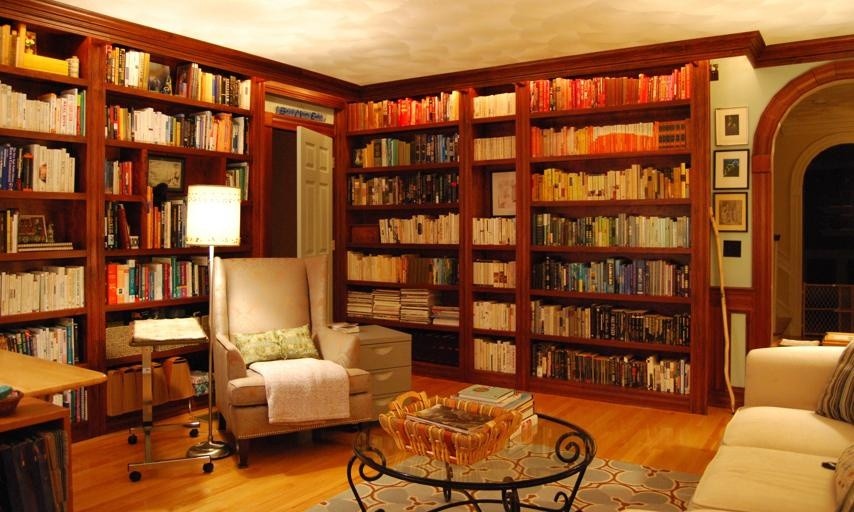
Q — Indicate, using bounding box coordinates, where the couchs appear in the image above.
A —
[682,346,852,512]
[201,257,373,468]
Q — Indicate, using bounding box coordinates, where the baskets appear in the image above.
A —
[378,390,523,465]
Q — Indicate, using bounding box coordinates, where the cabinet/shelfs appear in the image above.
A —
[1,15,107,434]
[109,44,270,432]
[338,325,412,421]
[340,95,465,387]
[522,73,707,395]
[467,88,522,392]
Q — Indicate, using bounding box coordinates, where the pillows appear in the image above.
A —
[235,323,322,366]
[813,339,854,421]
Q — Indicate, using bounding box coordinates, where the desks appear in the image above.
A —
[126,314,214,483]
[2,340,115,512]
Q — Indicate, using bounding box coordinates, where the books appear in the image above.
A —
[0,208,73,253]
[147,200,191,248]
[0,142,76,193]
[346,90,461,327]
[0,318,74,366]
[104,44,251,110]
[408,384,539,441]
[472,92,516,159]
[104,160,132,250]
[472,216,516,374]
[0,24,36,68]
[105,256,209,304]
[530,63,692,396]
[0,266,85,316]
[105,106,250,155]
[224,162,249,201]
[777,329,853,347]
[52,386,87,423]
[0,84,86,136]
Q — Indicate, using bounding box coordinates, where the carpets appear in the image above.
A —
[305,434,715,510]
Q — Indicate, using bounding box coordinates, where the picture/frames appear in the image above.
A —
[713,103,754,236]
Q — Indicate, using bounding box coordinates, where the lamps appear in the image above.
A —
[185,184,244,461]
